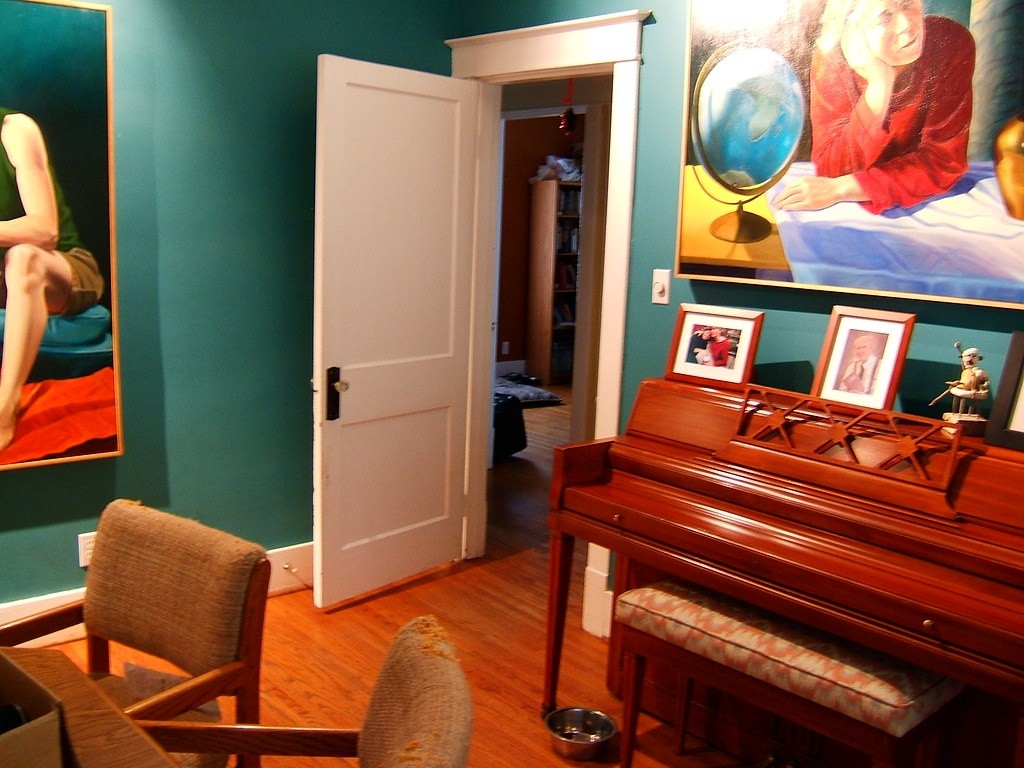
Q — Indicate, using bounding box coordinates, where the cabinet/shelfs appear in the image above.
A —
[530,177,585,385]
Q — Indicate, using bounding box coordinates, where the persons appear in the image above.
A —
[839,335,882,394]
[686,327,733,368]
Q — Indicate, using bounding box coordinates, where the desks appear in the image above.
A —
[0,646,180,768]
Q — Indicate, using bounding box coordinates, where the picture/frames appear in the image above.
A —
[0,0,124,471]
[664,303,765,392]
[673,0,1024,310]
[808,305,916,423]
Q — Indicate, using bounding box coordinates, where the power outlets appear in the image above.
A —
[502,342,508,354]
[78,532,97,567]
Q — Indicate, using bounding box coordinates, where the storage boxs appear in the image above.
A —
[0,651,81,768]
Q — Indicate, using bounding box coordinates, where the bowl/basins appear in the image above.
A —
[545,707,617,759]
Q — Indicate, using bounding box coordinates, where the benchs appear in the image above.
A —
[613,576,966,768]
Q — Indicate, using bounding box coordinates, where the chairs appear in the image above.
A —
[135,613,471,768]
[80,498,273,768]
